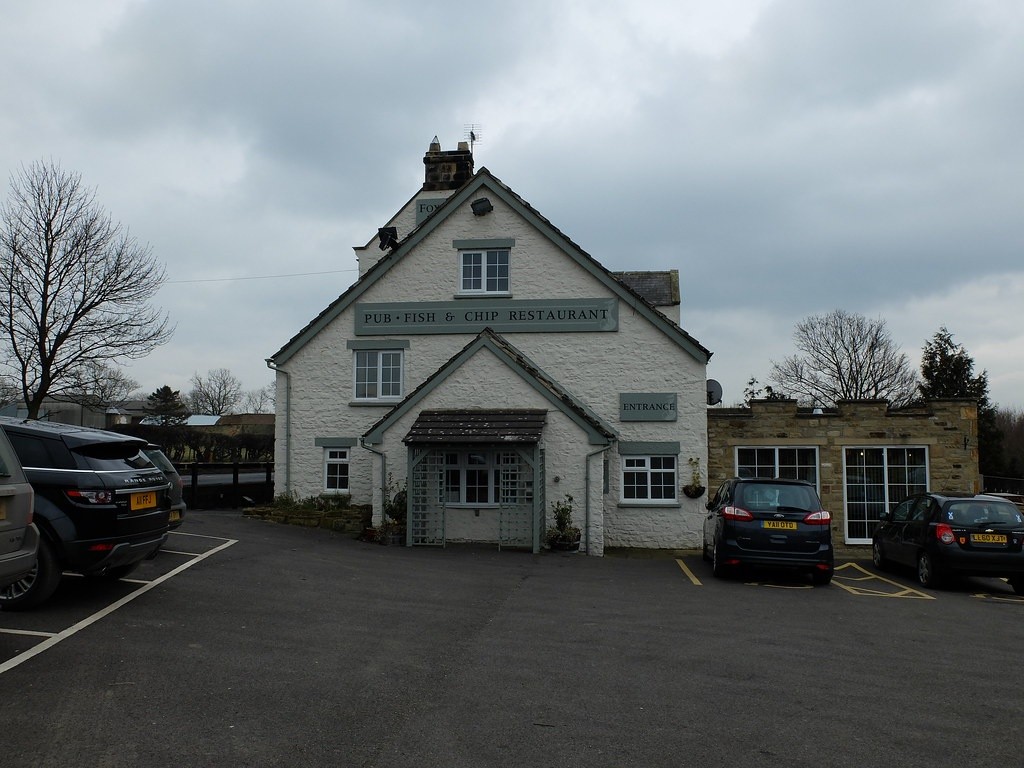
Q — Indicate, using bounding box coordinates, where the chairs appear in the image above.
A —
[967,505,986,520]
[949,510,963,519]
[778,493,791,505]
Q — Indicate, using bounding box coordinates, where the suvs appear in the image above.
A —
[702,476,836,587]
[0,414,188,610]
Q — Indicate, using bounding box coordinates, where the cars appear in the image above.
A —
[872,492,1024,597]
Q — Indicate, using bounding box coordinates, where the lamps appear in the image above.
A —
[470,198,493,217]
[378,226,397,252]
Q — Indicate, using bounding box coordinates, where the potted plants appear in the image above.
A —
[540,495,582,552]
[683,458,706,499]
[364,473,407,546]
